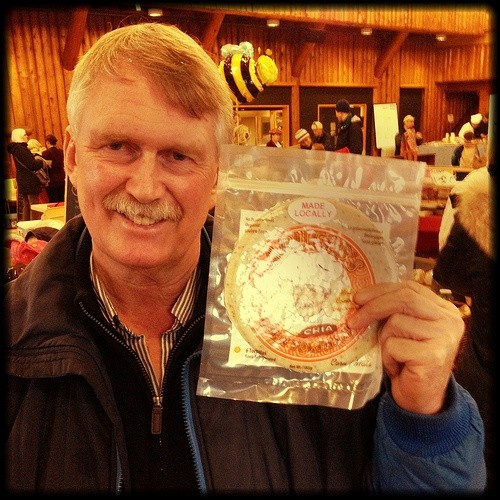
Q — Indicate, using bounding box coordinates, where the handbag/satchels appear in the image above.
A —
[35,168,50,188]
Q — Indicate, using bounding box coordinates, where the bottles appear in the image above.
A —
[444,132,455,143]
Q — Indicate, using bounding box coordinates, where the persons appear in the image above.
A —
[335,99,364,154]
[432,165,495,372]
[233,111,250,145]
[395,114,424,155]
[0,23,487,500]
[458,113,482,139]
[451,131,480,180]
[266,128,283,147]
[474,113,488,138]
[294,121,331,152]
[9,127,65,221]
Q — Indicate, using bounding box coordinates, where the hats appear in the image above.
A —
[336,99,350,112]
[294,129,309,144]
[269,128,282,135]
[471,113,482,125]
[311,121,323,130]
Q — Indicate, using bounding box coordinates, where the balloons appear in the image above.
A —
[216,43,278,105]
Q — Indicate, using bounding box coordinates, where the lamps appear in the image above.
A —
[267,19,280,27]
[360,28,373,36]
[147,8,164,17]
[436,33,448,42]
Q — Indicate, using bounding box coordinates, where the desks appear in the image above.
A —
[384,166,474,253]
[30,201,64,215]
[15,219,64,237]
